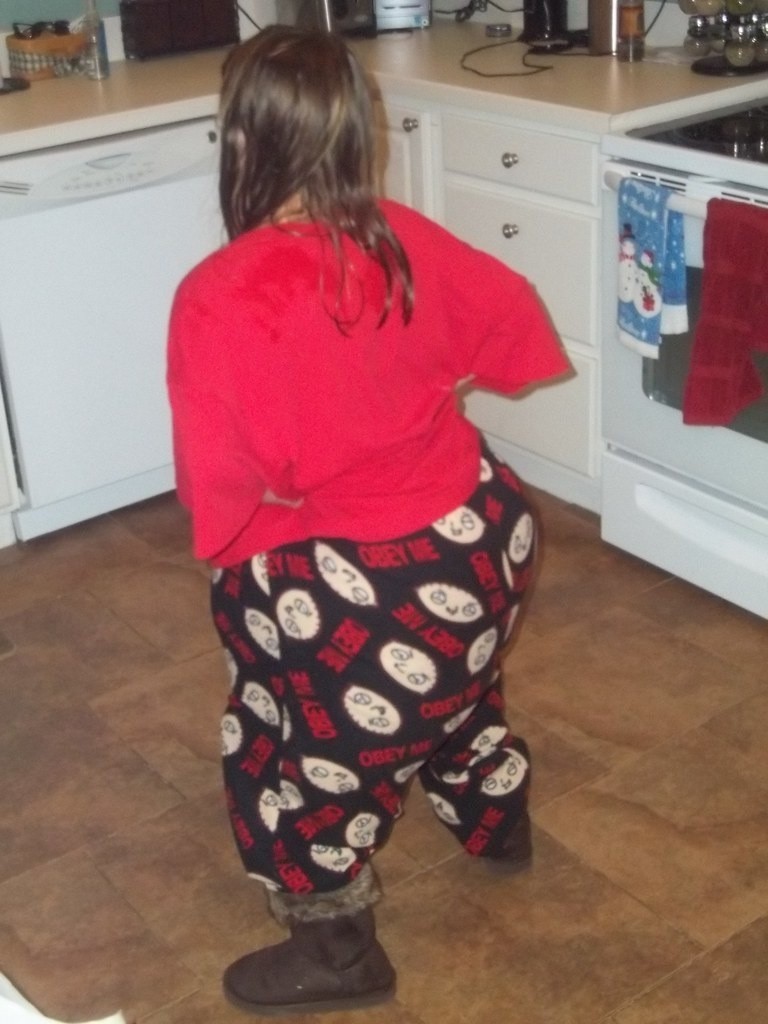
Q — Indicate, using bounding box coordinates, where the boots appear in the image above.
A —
[490,740,532,874]
[222,867,396,1008]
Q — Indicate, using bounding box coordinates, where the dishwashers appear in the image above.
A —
[589,160,766,624]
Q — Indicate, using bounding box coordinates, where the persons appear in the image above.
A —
[163,22,574,1014]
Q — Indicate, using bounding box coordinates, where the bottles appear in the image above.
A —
[82,0,109,81]
[588,0,646,62]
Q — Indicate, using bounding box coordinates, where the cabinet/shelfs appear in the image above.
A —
[378,102,429,215]
[428,121,609,478]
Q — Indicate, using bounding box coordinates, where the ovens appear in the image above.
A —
[0,118,236,544]
